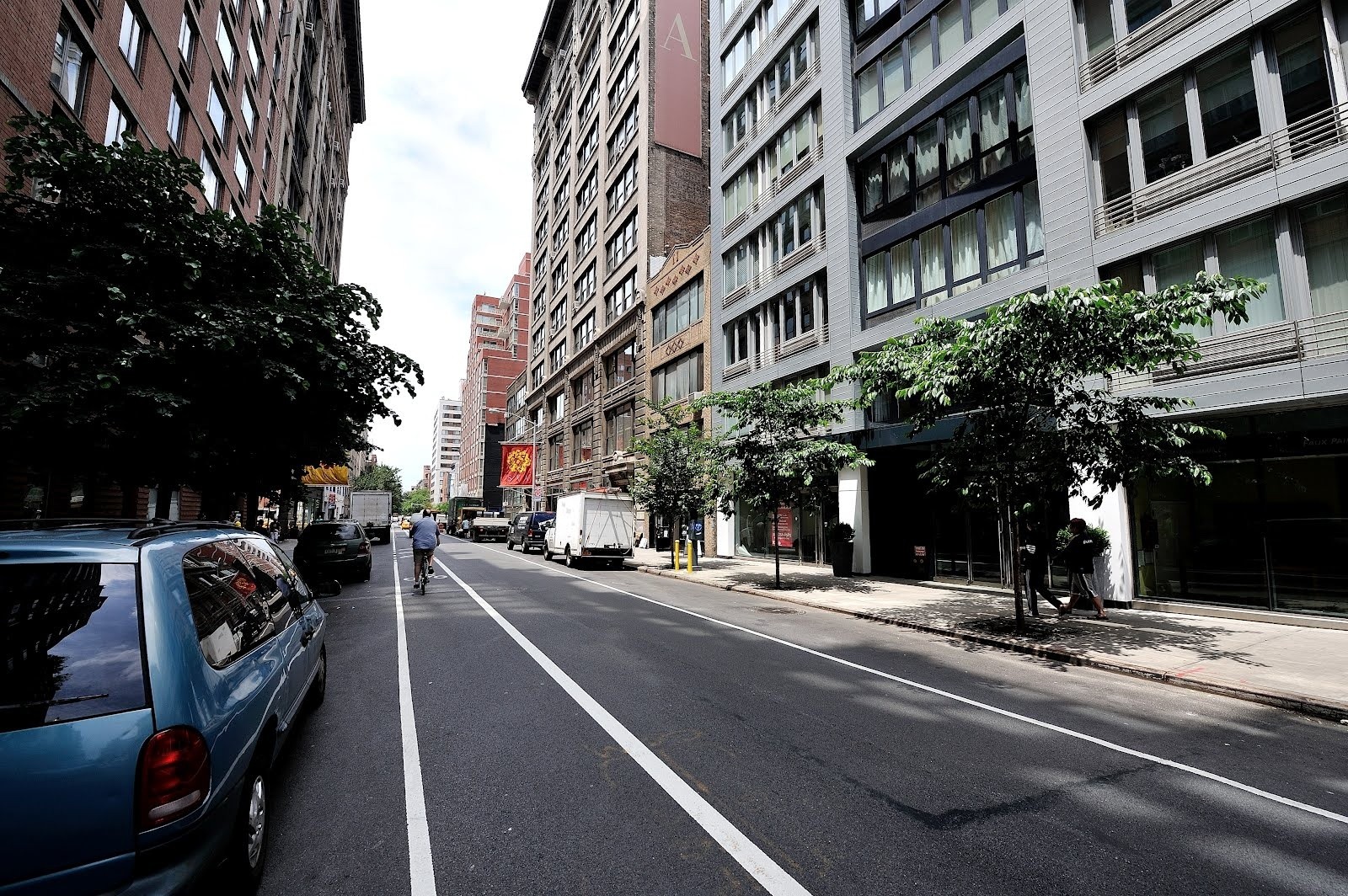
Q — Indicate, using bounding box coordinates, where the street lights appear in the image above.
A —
[487,409,536,511]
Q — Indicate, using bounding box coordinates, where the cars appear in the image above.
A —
[392,515,412,529]
[1,517,342,896]
[293,518,374,581]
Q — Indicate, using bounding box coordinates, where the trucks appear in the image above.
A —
[348,489,392,545]
[542,490,636,570]
[456,506,512,543]
[446,496,482,534]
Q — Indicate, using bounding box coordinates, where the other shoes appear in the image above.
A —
[413,583,420,589]
[1058,603,1067,620]
[428,565,434,575]
[1024,611,1040,620]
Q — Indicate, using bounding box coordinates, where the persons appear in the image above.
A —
[234,520,241,527]
[1055,519,1108,620]
[274,574,298,608]
[1019,517,1066,617]
[255,519,268,537]
[270,520,281,544]
[410,509,441,589]
[316,515,323,521]
[462,517,470,538]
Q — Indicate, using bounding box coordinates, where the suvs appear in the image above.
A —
[506,511,557,553]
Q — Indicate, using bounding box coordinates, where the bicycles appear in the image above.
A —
[408,535,440,596]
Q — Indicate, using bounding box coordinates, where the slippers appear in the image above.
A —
[1065,610,1072,614]
[1087,615,1108,620]
